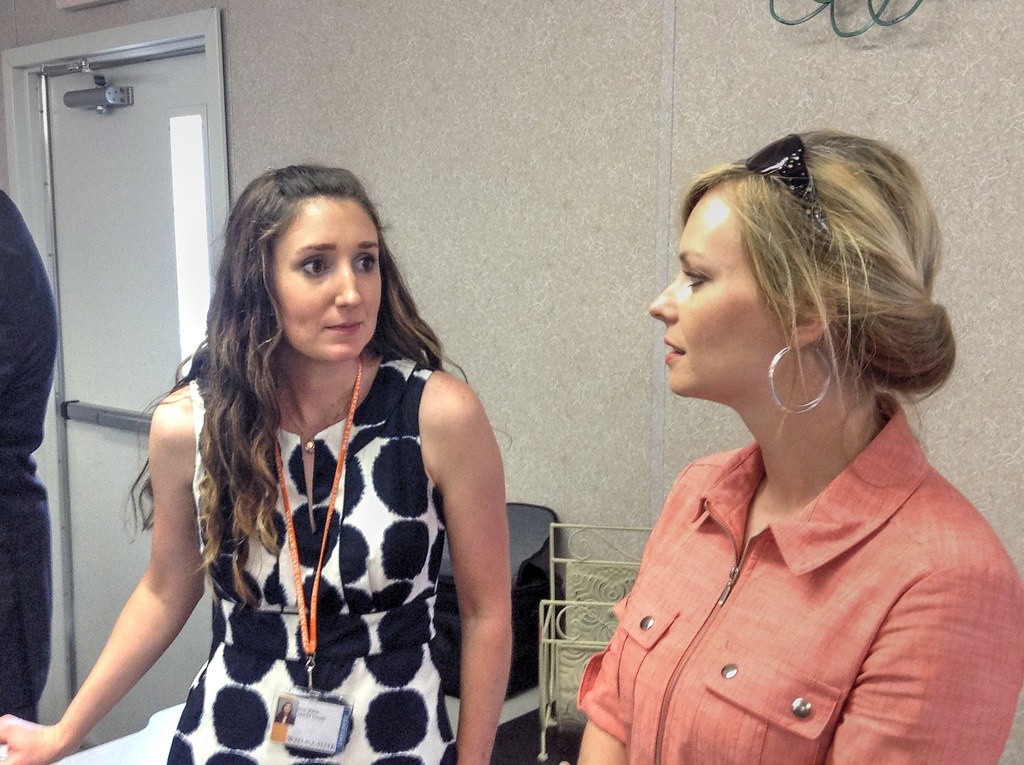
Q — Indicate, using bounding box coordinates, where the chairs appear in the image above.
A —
[429,503,564,742]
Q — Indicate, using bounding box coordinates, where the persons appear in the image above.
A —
[275,702,297,726]
[573,128,1024,765]
[0,162,514,763]
[0,188,60,729]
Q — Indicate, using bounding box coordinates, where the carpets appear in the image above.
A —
[489,701,588,765]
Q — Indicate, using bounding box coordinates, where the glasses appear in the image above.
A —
[745,135,837,266]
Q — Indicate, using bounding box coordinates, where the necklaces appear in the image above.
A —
[273,371,358,453]
